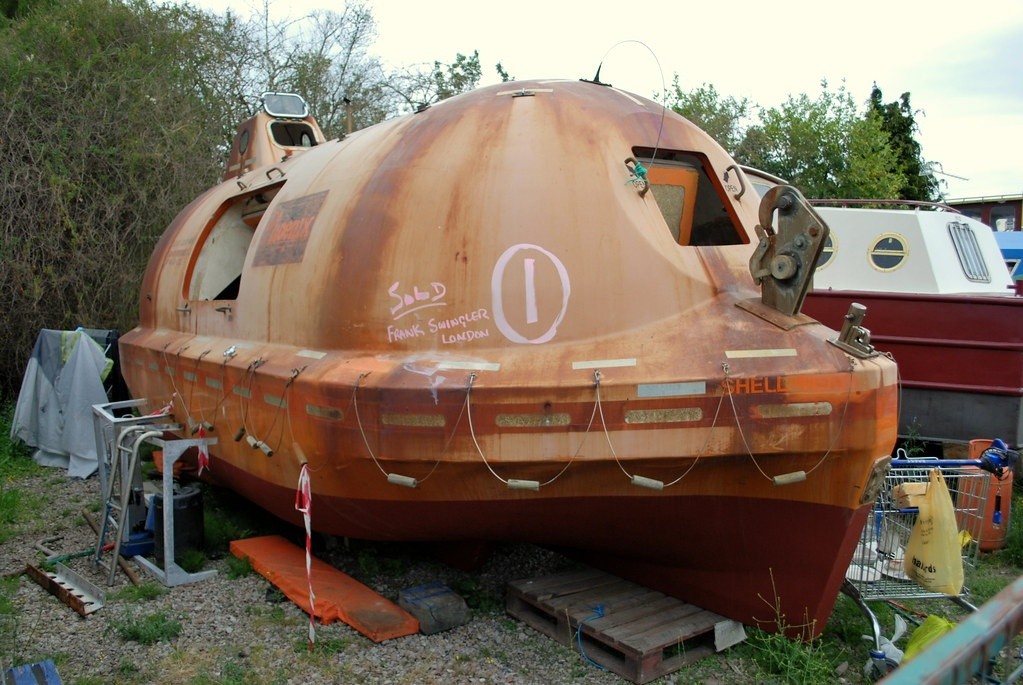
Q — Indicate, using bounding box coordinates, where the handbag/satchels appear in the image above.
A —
[861,614,905,675]
[904,469,964,596]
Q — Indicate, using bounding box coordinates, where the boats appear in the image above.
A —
[805,194,1023,452]
[118,41,897,643]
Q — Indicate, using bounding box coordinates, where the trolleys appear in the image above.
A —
[839,439,1020,685]
